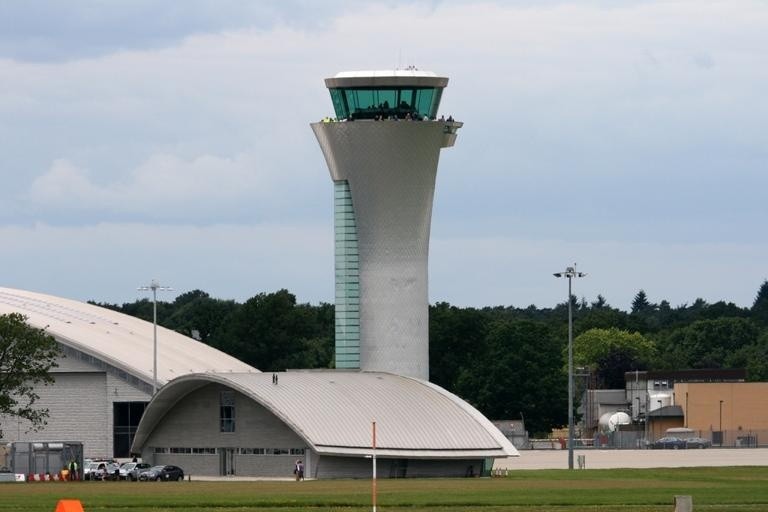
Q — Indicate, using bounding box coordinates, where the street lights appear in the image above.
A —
[657,400,661,438]
[137,281,171,397]
[552,263,587,473]
[718,400,723,446]
[635,397,641,449]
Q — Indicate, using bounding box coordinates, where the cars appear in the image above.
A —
[646,436,711,449]
[83,457,185,483]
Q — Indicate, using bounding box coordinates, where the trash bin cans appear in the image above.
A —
[577,454,586,469]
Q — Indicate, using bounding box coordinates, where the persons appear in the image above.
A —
[68,458,78,480]
[297,460,304,480]
